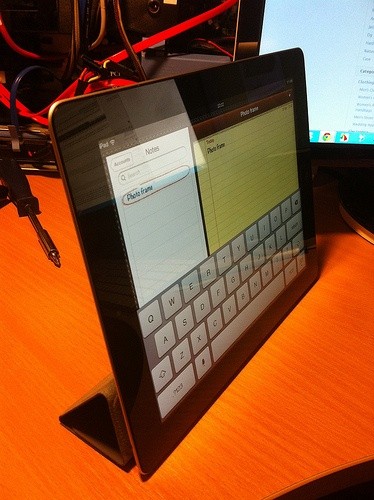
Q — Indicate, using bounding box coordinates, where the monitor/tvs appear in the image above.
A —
[231,0,373,167]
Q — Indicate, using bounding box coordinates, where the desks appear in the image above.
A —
[1,161,374,498]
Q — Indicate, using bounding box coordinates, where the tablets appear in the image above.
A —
[48,47,319,477]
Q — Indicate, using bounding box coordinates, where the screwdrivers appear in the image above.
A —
[2,161,62,268]
